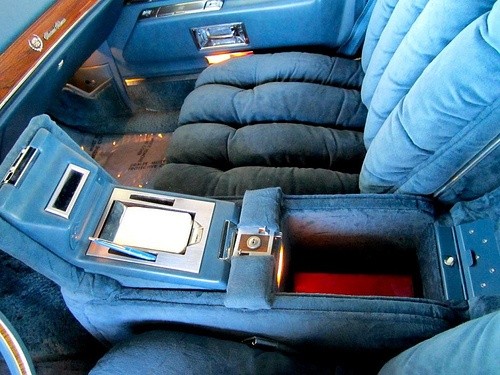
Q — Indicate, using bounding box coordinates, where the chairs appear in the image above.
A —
[153,0,500,205]
[84,313,500,375]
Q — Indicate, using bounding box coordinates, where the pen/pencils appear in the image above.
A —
[89,237,157,260]
